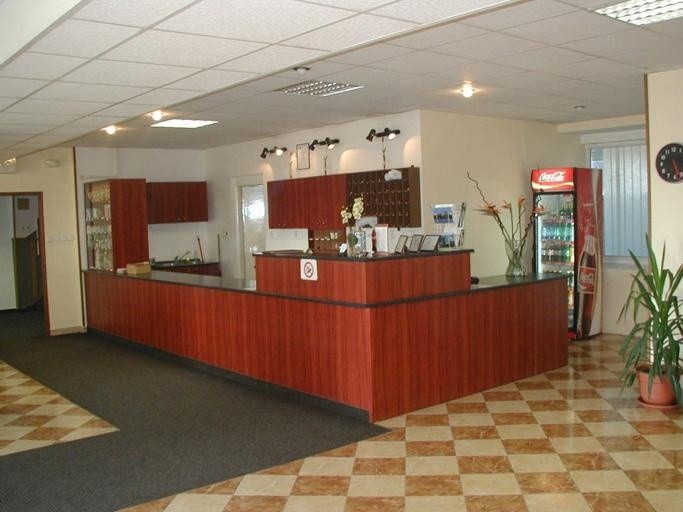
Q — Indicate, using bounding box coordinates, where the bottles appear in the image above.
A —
[538,206,573,289]
[87,231,112,271]
[566,289,573,328]
[572,225,598,338]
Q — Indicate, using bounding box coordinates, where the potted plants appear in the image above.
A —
[612,230,683,412]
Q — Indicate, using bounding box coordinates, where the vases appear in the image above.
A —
[503,238,526,279]
[346,229,360,257]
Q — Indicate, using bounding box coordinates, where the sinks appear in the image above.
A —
[154,260,200,267]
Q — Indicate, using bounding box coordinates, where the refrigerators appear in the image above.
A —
[526,166,605,340]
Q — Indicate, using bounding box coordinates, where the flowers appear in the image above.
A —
[342,194,367,228]
[463,169,549,238]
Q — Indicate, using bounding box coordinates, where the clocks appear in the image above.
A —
[655,141,683,184]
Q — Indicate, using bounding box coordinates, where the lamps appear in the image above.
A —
[260,145,286,164]
[307,137,339,180]
[365,127,399,172]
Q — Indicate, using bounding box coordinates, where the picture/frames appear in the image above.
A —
[296,143,310,169]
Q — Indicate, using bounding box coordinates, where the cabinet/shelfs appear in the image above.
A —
[346,166,421,230]
[305,175,346,229]
[85,178,150,272]
[266,178,306,229]
[147,181,209,224]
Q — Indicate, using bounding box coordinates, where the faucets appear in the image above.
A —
[177,250,191,261]
